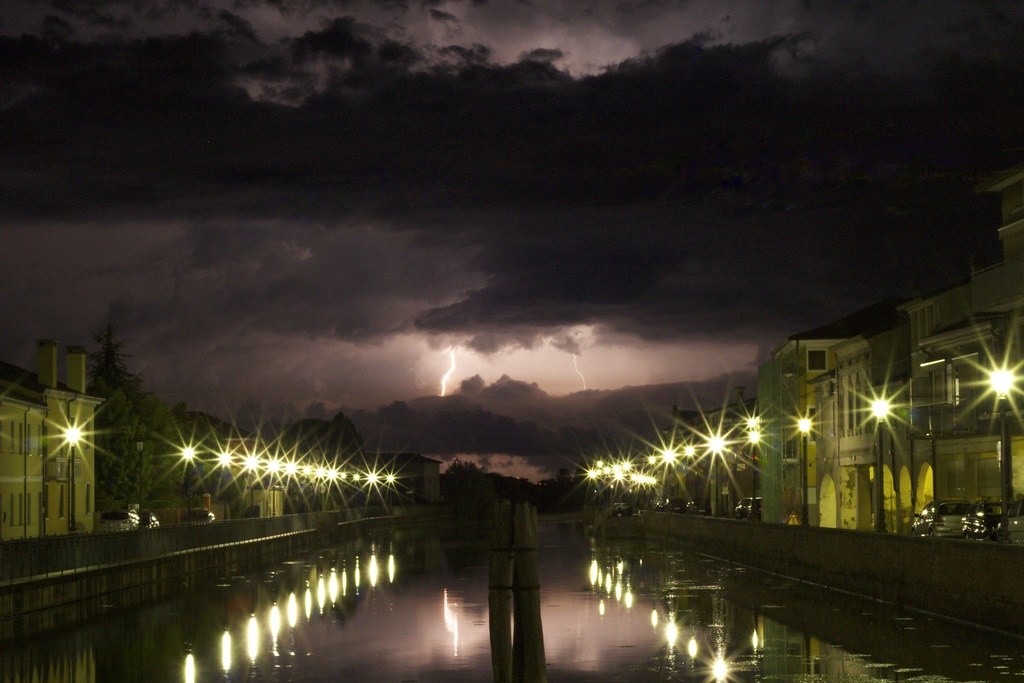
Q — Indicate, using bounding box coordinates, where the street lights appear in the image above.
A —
[586,359,1014,542]
[64,419,395,530]
